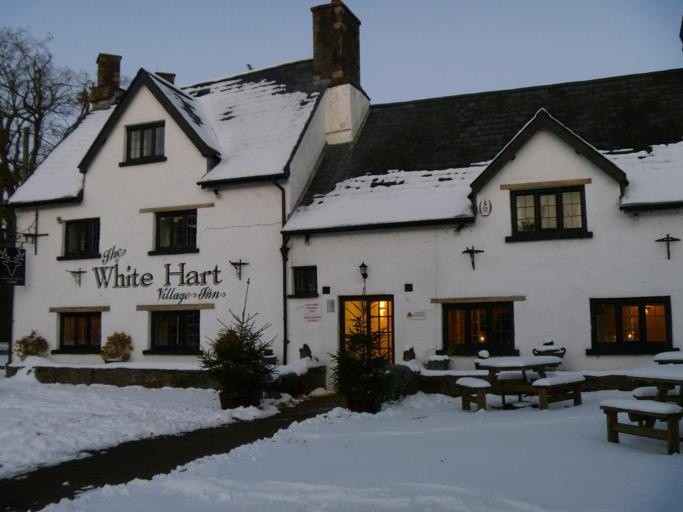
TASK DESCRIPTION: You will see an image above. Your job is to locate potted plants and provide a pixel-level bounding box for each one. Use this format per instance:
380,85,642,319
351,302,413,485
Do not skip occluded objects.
99,332,133,363
13,331,47,361
203,308,278,411
329,325,386,417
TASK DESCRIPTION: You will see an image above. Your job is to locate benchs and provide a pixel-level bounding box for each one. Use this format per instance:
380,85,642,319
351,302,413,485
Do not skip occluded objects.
455,371,585,411
600,387,683,455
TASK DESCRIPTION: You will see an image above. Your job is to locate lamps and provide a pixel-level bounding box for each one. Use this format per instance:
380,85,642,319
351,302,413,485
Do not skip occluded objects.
358,261,367,279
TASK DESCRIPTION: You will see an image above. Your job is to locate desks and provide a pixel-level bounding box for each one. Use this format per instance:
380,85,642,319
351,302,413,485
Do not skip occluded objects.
625,363,683,403
474,356,563,384
